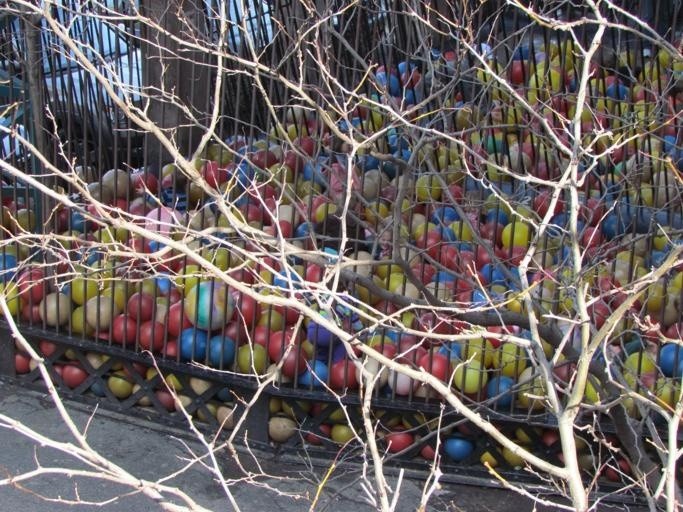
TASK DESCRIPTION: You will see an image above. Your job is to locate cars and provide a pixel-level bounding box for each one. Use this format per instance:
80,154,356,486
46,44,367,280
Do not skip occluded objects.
0,0,391,180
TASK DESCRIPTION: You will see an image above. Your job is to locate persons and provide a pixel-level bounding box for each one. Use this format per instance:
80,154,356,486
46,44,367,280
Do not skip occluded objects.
146,171,189,213
317,212,366,257
